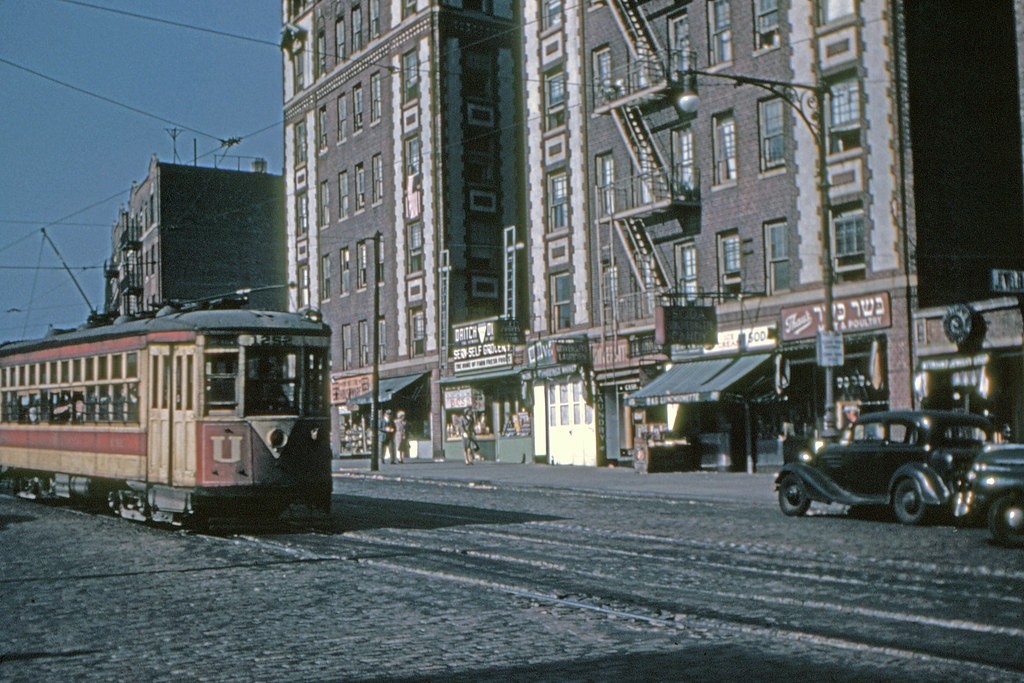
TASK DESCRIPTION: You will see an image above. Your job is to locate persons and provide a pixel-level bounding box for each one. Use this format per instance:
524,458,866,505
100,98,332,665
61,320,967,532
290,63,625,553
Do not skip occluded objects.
378,409,398,465
459,407,478,465
392,411,408,465
1,379,297,425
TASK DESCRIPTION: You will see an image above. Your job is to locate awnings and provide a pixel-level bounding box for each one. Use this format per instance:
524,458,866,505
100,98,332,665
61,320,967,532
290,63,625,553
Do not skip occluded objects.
345,370,431,405
623,351,775,407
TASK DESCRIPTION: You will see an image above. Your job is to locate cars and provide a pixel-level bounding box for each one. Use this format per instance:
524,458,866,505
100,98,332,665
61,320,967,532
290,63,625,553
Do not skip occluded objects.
952,444,1024,548
775,408,1002,526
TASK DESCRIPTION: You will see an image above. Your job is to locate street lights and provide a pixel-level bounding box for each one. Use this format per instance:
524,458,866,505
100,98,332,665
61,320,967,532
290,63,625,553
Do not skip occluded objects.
670,66,842,449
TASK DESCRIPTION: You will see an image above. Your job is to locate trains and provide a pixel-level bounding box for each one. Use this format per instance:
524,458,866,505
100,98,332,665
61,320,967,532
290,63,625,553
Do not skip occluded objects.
0,283,335,531
